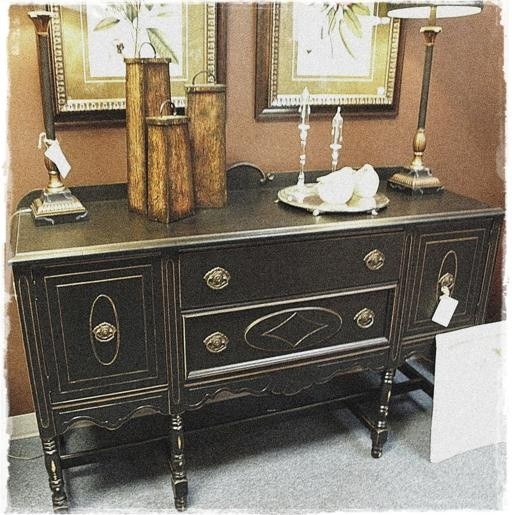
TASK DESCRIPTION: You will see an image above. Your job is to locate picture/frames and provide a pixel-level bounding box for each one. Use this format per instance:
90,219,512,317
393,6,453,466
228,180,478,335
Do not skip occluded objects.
253,1,408,123
32,0,228,130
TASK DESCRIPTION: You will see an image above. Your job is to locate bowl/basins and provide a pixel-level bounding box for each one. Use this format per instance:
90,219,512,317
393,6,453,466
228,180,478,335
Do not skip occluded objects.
316,164,379,205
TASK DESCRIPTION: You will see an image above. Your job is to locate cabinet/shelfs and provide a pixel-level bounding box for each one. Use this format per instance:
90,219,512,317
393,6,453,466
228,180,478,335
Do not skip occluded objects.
7,161,505,514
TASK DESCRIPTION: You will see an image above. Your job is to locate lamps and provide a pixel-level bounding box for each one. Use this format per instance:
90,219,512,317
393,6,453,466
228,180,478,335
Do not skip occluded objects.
29,5,89,227
386,0,483,195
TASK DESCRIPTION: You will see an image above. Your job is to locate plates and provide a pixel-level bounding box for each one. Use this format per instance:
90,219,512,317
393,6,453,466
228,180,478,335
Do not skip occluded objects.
276,183,390,215
